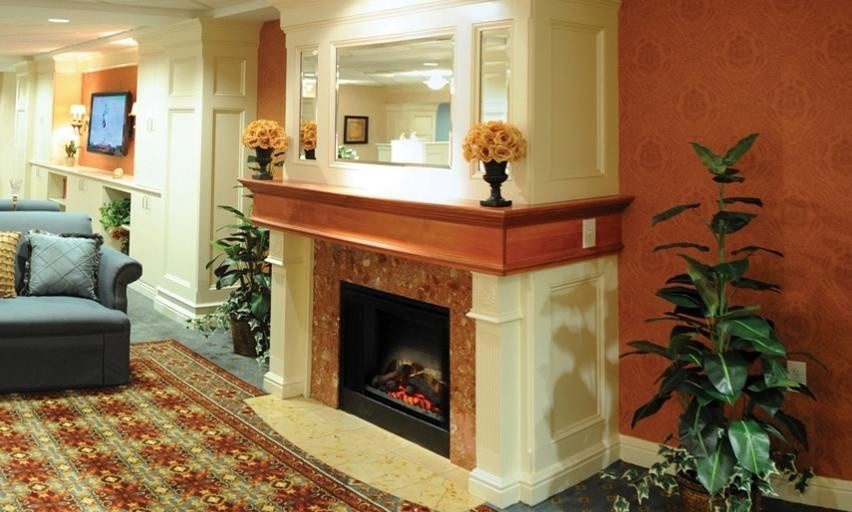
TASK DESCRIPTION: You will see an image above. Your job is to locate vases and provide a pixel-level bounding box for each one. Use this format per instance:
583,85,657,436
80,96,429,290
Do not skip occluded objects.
253,146,274,179
480,160,513,207
304,149,316,160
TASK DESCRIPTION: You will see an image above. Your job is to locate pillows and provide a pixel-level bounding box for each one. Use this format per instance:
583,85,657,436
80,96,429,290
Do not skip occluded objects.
0,229,106,302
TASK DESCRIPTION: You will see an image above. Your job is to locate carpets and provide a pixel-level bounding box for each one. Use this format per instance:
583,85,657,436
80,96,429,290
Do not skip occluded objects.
1,338,501,512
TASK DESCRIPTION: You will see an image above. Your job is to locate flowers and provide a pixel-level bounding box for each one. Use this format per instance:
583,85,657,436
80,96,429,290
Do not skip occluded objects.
238,118,289,150
300,119,317,151
462,119,527,163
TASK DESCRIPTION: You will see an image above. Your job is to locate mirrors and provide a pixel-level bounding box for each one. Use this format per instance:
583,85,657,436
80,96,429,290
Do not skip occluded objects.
474,26,512,177
298,47,319,161
336,34,451,168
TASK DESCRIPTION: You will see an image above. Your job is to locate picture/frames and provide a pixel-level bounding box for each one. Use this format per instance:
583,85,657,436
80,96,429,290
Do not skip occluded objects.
343,115,370,145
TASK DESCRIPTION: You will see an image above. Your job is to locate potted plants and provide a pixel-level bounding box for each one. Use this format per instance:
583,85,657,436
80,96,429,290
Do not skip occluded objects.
594,113,831,512
182,152,287,372
64,140,82,166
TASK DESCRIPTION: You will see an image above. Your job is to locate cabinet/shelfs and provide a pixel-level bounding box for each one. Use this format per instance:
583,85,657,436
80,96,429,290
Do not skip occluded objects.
29,164,65,201
33,53,82,162
64,174,125,254
129,189,169,303
132,25,172,190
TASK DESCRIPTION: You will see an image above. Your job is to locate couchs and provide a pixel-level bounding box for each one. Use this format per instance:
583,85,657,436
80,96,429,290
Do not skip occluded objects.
0,211,143,392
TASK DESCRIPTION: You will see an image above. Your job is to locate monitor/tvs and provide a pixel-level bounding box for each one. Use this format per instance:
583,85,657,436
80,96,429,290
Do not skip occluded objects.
87,92,131,156
